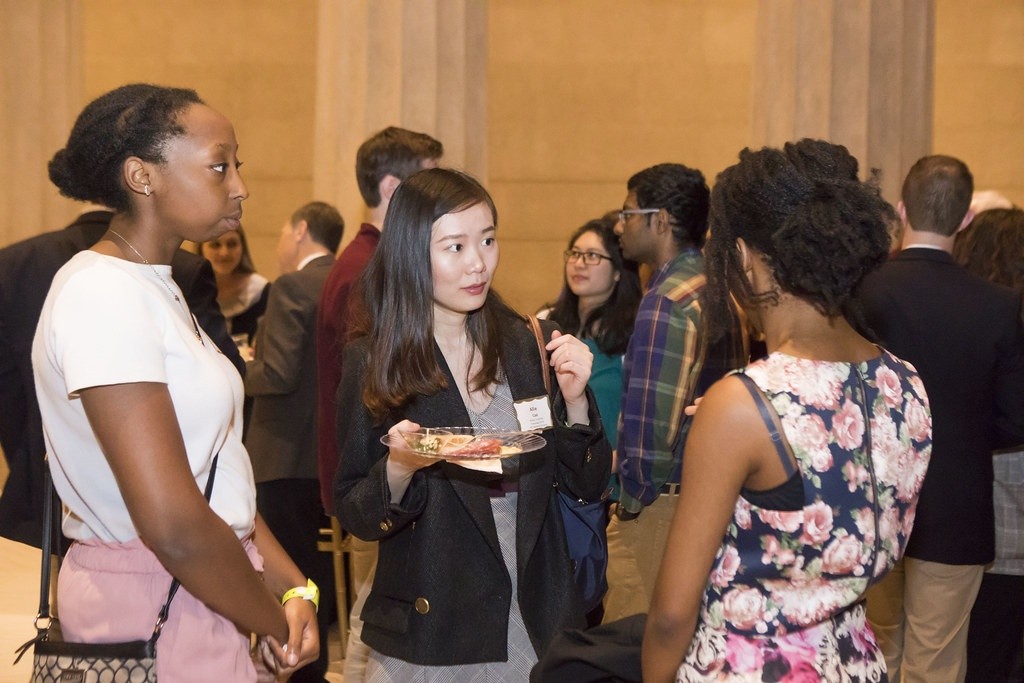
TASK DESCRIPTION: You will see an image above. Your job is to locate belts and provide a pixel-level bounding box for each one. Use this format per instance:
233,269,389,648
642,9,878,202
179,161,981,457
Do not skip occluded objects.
660,485,680,494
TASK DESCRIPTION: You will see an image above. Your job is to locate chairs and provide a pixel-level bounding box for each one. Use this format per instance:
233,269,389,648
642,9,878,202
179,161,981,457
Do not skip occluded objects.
316,511,378,661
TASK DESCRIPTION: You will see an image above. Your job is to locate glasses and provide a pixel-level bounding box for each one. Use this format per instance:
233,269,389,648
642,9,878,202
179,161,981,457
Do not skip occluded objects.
617,209,660,224
561,250,612,265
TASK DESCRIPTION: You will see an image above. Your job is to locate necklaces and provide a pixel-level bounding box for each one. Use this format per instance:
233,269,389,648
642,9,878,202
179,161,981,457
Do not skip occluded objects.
106,223,206,346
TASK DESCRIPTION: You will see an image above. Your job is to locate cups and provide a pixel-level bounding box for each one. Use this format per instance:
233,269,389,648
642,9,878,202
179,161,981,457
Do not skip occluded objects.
231,333,249,350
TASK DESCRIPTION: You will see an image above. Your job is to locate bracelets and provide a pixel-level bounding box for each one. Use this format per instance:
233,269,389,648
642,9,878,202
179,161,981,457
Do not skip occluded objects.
280,578,321,613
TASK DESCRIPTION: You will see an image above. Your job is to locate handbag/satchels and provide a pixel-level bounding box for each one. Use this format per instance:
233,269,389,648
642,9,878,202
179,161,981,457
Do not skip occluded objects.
12,630,158,683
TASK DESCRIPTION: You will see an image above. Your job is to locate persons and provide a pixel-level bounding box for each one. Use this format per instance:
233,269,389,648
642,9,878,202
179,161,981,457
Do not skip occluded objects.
535,137,1024,683
331,165,613,683
32,82,322,683
315,125,443,683
245,201,353,683
0,210,246,558
196,218,272,347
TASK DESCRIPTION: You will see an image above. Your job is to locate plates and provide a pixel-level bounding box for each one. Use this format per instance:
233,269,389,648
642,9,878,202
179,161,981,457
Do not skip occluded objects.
380,427,547,459
